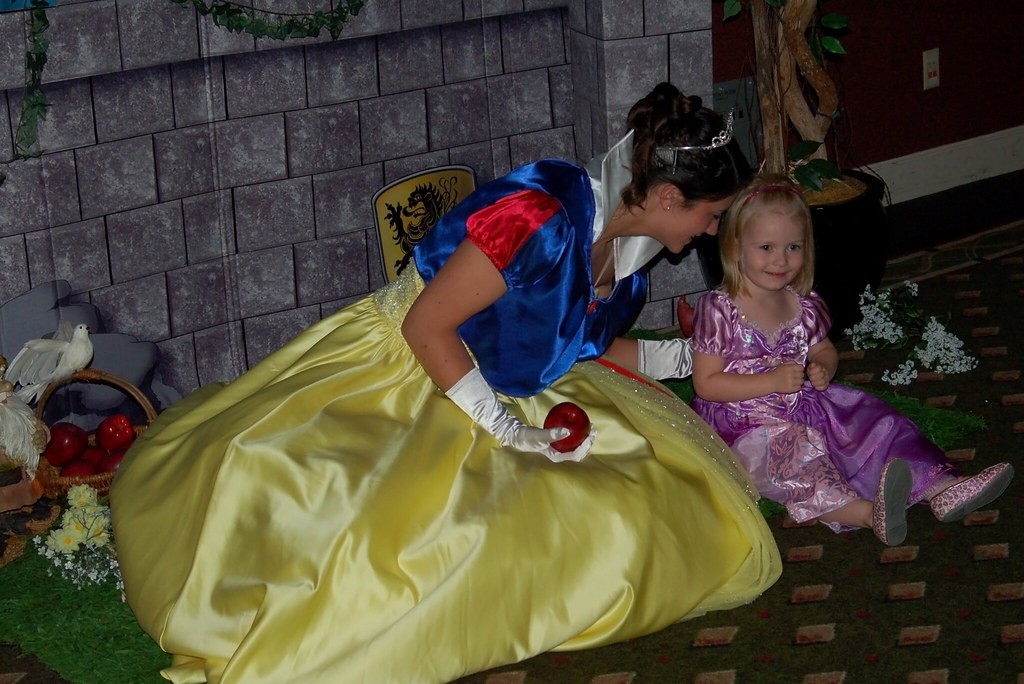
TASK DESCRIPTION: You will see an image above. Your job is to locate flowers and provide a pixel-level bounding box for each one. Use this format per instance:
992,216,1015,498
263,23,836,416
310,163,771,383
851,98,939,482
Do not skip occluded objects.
32,483,137,602
843,278,982,389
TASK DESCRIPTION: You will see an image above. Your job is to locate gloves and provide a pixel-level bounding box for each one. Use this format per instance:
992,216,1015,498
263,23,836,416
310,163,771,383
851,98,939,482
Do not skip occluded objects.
638,338,695,380
444,368,597,463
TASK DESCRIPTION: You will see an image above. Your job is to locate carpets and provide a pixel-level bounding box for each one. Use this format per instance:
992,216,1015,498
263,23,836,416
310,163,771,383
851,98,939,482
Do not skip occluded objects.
0,329,987,684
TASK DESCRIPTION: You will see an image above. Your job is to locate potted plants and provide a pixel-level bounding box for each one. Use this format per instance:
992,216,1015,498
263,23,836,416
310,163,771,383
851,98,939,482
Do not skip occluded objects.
691,0,886,343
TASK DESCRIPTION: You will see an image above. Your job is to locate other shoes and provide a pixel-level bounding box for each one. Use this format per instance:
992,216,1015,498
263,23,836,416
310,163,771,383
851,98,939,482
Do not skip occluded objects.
930,462,1013,522
872,458,913,547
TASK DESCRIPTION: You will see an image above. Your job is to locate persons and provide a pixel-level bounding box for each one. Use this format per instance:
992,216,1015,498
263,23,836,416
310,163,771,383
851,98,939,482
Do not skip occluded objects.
691,173,1015,547
108,80,784,684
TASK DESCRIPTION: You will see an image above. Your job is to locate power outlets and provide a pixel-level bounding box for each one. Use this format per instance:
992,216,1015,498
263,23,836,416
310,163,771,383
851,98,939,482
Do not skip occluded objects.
922,47,940,91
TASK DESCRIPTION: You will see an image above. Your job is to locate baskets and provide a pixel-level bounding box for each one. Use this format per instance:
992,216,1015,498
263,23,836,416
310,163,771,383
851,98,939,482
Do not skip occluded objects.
32,369,157,500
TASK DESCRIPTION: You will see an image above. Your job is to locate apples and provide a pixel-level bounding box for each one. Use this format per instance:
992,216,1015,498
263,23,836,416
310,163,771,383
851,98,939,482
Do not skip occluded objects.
543,402,591,453
44,415,133,477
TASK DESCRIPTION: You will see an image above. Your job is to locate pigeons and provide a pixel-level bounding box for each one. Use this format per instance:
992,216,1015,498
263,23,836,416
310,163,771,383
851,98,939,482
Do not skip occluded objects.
4,319,94,404
